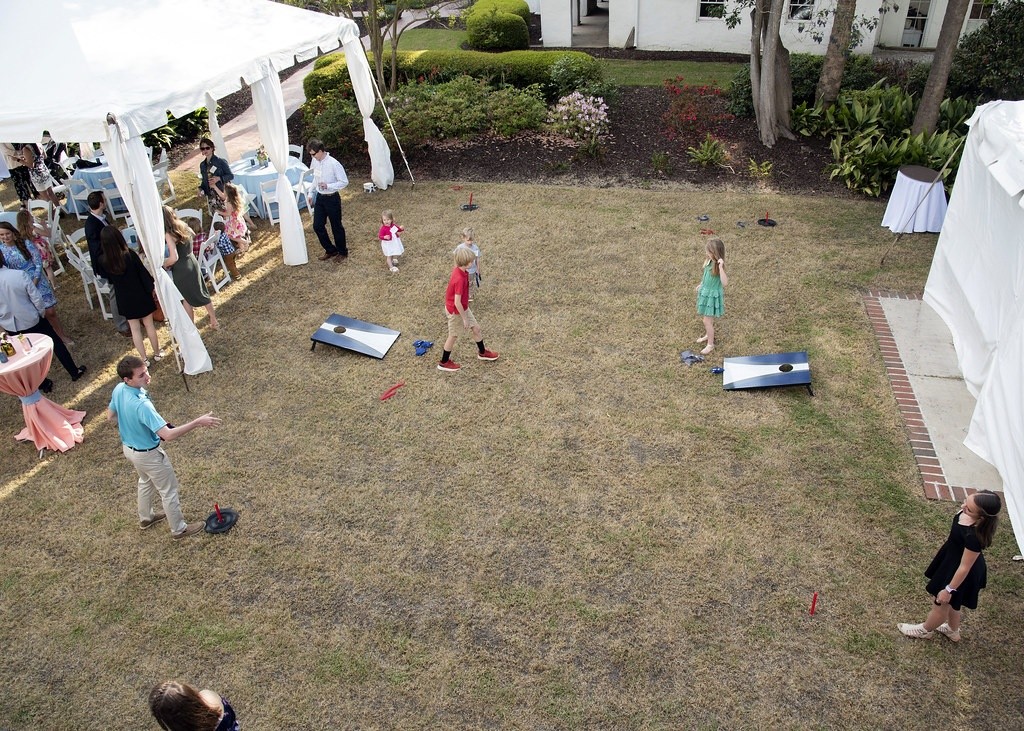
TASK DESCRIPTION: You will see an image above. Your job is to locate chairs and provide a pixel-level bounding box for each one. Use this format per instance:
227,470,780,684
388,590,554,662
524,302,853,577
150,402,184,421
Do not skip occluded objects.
25,145,311,320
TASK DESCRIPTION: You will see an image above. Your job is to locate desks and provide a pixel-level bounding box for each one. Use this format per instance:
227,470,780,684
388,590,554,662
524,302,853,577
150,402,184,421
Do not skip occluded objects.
0,334,87,463
226,155,315,220
126,240,173,289
880,166,948,233
63,156,126,214
0,211,22,232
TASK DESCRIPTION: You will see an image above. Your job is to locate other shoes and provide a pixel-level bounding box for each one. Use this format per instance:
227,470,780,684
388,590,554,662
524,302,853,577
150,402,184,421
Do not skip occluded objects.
388,265,399,273
61,337,76,346
154,350,164,363
119,328,132,337
209,323,220,331
700,344,714,355
468,294,475,302
72,365,86,381
144,359,150,368
697,336,708,343
393,259,399,264
44,379,53,394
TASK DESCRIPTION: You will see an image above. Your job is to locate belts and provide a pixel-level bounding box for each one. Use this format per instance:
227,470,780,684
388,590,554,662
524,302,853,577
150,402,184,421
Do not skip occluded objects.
318,193,335,196
128,443,160,452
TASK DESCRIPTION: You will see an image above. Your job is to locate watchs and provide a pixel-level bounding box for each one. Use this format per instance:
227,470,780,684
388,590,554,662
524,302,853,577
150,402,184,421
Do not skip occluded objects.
946,585,957,594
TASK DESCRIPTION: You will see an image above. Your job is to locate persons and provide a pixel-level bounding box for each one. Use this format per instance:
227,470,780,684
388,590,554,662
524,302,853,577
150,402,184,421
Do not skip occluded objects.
98,226,166,367
197,138,235,216
896,490,1001,643
0,143,60,217
107,355,222,541
188,217,210,275
695,238,728,355
162,204,220,330
213,222,242,280
456,228,482,301
0,220,75,345
378,210,405,271
85,190,144,337
148,680,238,731
209,183,251,254
437,246,500,371
0,250,87,393
16,210,58,292
307,139,350,261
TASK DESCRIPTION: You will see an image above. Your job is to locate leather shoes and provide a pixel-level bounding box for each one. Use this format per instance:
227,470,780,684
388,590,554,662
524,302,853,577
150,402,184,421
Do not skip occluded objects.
333,251,348,263
318,250,337,259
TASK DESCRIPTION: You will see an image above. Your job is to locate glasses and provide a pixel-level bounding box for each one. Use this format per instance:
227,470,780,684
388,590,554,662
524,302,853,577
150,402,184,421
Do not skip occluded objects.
309,151,318,155
200,146,211,150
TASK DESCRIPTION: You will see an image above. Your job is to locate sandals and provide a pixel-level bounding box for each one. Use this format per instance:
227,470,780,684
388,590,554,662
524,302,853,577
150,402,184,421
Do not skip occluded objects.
897,623,932,640
936,622,960,642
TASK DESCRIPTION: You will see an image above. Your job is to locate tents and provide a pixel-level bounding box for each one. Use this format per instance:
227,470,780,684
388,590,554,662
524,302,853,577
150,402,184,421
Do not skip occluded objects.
918,100,1024,562
0,0,395,377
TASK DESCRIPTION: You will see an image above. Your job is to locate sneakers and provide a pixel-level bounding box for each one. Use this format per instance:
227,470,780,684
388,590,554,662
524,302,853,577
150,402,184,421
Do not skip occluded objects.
173,521,206,541
478,350,499,361
437,359,461,372
140,513,166,529
